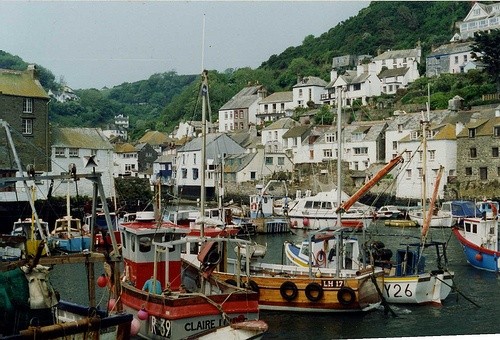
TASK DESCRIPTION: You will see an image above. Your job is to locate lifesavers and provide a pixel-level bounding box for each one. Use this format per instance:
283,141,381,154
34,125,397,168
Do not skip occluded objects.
337,287,356,306
280,281,298,301
283,209,288,217
221,280,260,297
305,282,323,302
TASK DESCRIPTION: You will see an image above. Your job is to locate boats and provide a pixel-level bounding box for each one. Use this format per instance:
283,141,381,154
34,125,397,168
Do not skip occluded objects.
0,14,499,340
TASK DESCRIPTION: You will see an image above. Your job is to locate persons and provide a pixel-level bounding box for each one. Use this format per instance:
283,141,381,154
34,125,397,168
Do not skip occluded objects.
142,276,162,295
